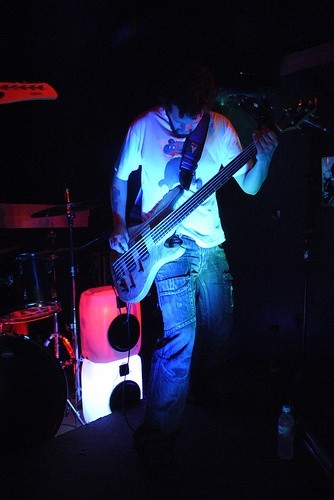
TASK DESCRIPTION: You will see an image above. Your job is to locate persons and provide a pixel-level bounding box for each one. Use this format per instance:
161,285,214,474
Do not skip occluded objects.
108,59,280,447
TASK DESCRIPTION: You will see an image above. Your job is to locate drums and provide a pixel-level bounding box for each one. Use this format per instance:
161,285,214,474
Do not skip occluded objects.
1,330,69,473
1,302,69,360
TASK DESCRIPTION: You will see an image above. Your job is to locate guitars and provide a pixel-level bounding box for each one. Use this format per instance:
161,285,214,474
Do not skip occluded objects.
0,81,60,107
110,96,320,301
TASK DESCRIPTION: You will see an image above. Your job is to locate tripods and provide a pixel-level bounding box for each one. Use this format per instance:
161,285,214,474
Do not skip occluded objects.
44,228,74,360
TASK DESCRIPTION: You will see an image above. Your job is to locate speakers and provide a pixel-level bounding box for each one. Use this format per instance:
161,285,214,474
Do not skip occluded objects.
80,355,143,425
80,285,141,363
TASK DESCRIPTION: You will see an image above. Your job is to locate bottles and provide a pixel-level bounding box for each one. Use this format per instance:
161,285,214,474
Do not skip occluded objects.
277,406,295,461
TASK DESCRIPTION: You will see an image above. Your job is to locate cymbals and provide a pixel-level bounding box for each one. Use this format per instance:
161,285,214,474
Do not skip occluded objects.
12,244,89,260
31,200,105,218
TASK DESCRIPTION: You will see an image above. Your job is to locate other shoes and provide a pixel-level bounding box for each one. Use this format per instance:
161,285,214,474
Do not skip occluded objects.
137,425,185,470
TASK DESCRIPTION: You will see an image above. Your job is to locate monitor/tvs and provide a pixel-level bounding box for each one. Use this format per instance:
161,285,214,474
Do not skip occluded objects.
310,148,334,210
